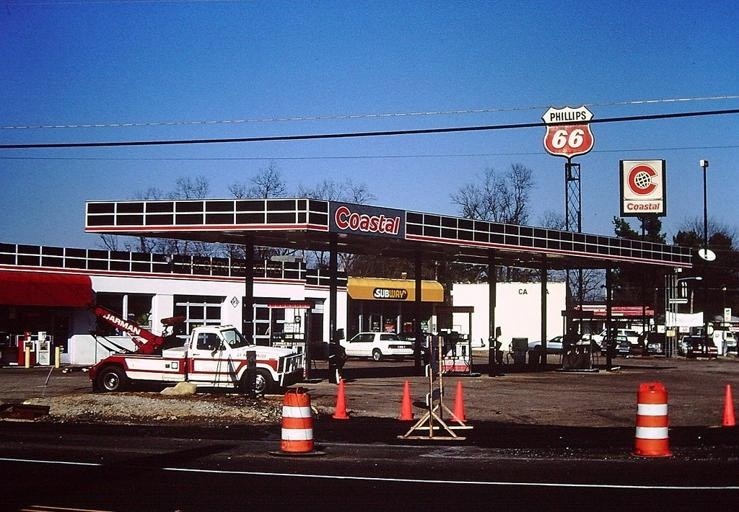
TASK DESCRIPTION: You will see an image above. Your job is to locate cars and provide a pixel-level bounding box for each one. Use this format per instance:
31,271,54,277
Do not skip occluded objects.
339,330,415,362
526,326,739,360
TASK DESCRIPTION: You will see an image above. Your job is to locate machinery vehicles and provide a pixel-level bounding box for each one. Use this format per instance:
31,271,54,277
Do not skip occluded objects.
81,302,306,391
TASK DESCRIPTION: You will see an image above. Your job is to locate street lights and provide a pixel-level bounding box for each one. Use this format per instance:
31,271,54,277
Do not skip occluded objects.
698,158,712,354
674,276,703,355
721,286,728,307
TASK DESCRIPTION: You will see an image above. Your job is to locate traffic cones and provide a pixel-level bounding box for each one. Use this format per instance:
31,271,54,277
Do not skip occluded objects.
329,377,350,420
397,380,415,421
448,379,469,424
717,382,738,428
